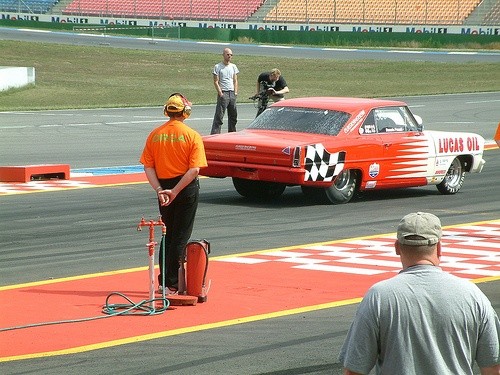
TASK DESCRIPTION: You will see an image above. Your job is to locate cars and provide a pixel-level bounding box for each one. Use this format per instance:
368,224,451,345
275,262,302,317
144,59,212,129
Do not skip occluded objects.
196,95,485,206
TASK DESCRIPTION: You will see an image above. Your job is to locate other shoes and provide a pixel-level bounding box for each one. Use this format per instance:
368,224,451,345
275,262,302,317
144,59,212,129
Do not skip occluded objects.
158,285,175,295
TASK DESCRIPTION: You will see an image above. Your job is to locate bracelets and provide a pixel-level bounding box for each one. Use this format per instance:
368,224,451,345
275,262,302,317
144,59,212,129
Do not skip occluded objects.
155,187,162,191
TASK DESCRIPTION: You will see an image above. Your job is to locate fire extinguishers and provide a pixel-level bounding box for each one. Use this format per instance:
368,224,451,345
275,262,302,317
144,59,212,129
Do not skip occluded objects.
182,239,211,302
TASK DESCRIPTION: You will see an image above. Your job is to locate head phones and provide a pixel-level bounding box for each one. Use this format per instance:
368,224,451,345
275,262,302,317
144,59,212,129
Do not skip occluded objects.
164,93,191,118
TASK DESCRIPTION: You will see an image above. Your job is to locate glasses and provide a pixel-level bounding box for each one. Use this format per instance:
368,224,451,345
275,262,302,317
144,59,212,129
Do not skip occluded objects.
227,54,232,56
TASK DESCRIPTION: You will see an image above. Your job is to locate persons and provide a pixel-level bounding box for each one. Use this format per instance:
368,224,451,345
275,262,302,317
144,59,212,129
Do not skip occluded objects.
210,47,239,136
338,212,500,375
255,69,289,119
140,94,208,295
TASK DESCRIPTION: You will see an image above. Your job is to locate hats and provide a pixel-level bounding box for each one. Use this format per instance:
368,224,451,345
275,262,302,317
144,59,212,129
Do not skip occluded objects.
167,95,192,113
397,212,441,246
